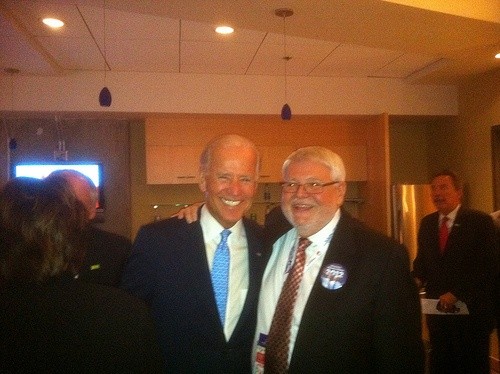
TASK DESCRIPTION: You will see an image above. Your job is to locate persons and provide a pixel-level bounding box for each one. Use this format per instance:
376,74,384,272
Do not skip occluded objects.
0,170,160,374
171,146,425,374
116,134,276,374
409,170,500,374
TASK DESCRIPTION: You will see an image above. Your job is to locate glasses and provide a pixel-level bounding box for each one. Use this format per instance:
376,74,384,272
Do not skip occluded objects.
279,179,340,194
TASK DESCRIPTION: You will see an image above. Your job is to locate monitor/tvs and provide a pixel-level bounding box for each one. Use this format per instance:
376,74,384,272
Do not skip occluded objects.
11,159,106,221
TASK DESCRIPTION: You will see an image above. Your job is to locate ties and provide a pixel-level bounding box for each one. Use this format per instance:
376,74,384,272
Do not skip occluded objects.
210,228,231,331
439,216,449,255
262,237,312,374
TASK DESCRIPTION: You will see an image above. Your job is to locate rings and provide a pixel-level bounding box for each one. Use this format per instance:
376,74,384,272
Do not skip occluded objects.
443,305,449,309
